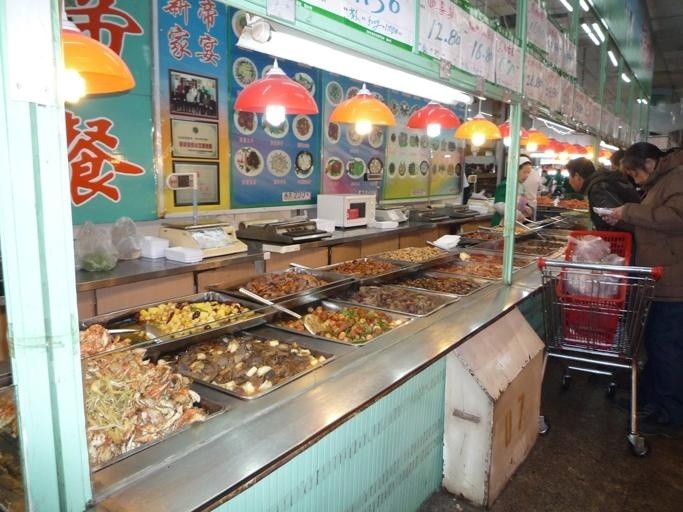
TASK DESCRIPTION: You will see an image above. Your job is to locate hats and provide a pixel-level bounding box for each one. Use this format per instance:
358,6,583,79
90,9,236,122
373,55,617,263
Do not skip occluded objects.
517,155,532,166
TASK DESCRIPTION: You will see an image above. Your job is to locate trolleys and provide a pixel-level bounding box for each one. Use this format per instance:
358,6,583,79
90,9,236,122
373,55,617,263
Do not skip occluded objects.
536,255,663,458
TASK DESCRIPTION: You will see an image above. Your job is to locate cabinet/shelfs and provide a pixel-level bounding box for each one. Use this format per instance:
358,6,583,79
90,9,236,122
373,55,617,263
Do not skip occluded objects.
2,220,492,388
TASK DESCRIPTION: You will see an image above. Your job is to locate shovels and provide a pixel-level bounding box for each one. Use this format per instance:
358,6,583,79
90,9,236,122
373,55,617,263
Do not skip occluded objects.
426,240,471,261
239,288,326,337
515,219,546,240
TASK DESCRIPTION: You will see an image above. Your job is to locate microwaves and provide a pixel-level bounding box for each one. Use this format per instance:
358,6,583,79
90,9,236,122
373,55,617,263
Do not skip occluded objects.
316,194,376,228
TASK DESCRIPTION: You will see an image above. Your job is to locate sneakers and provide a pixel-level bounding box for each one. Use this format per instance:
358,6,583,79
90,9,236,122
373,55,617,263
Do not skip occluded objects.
603,384,681,440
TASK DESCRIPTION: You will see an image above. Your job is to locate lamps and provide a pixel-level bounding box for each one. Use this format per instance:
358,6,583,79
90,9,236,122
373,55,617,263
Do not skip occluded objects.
329,83,394,135
235,58,319,127
454,96,503,146
235,13,476,105
408,100,461,138
497,115,620,166
61,0,135,104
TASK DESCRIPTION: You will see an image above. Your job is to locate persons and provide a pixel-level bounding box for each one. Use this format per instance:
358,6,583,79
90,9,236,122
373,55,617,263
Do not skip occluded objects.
542,169,553,196
519,153,541,222
608,149,647,199
597,139,682,441
488,154,533,228
564,156,642,388
554,167,563,196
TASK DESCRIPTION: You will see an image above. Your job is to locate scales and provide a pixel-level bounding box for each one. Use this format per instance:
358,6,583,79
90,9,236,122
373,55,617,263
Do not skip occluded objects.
160,172,249,259
